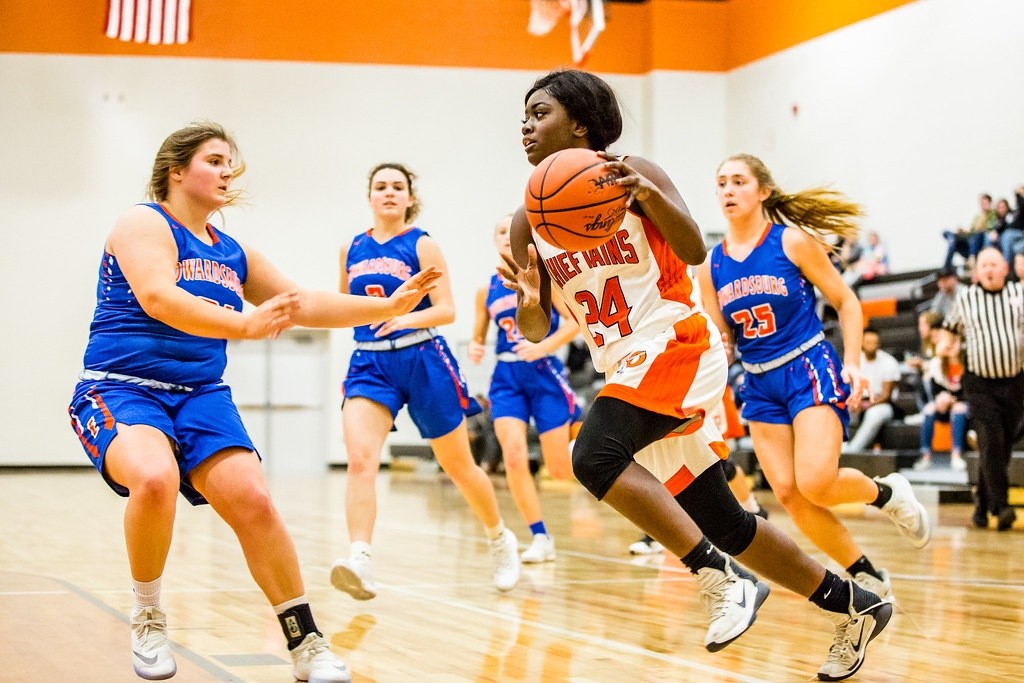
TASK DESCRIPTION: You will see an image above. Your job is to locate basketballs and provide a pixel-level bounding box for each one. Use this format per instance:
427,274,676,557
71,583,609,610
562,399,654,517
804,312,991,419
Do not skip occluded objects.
524,147,628,253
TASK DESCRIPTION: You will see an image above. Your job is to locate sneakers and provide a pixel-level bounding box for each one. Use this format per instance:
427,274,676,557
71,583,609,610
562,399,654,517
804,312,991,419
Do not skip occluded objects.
873,472,930,548
130,607,177,680
487,529,520,591
817,580,892,681
851,568,896,603
330,549,376,600
288,631,349,683
690,552,770,652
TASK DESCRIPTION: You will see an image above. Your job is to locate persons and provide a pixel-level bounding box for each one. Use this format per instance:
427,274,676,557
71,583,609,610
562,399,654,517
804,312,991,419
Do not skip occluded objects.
328,163,521,600
468,186,1023,556
698,155,930,602
69,126,443,683
467,214,577,564
494,71,893,681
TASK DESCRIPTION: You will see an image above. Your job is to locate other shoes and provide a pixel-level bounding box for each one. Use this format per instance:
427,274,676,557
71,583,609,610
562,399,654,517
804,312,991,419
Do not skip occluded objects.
997,507,1016,530
973,492,988,527
522,533,554,563
630,534,665,555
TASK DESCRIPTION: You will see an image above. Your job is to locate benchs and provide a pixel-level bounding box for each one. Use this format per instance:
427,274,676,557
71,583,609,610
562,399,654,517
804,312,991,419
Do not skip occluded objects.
840,453,919,482
390,446,436,471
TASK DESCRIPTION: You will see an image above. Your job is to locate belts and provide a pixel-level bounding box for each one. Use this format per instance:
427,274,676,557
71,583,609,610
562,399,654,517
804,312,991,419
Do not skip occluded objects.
741,330,825,374
496,351,523,362
354,327,438,351
